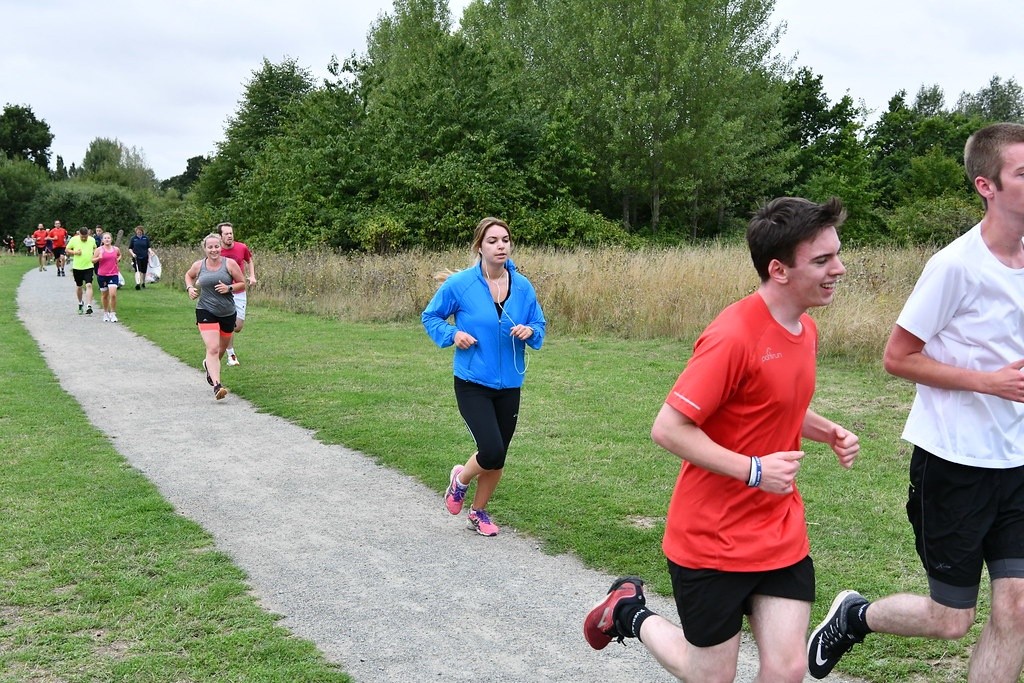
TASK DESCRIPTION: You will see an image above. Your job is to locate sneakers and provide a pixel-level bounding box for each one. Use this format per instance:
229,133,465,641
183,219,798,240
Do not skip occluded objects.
78,299,83,315
807,589,869,680
103,311,108,322
109,311,118,323
444,463,468,515
584,573,646,649
226,355,240,367
467,502,498,537
203,359,216,386
86,307,93,315
214,382,227,401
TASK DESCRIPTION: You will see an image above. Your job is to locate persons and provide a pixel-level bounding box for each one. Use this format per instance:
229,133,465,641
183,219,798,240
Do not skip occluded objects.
65,227,98,315
45,229,53,263
421,217,547,536
47,219,68,276
4,235,14,254
30,235,36,255
217,222,256,366
94,225,104,275
66,235,71,265
32,224,47,272
24,235,32,256
129,227,153,289
185,234,246,400
92,233,122,322
807,121,1024,683
88,228,93,237
583,196,859,683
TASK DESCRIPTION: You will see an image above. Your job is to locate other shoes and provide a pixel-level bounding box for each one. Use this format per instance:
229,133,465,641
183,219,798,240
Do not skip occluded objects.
42,266,48,272
135,284,141,290
39,265,44,273
61,270,65,277
58,270,61,278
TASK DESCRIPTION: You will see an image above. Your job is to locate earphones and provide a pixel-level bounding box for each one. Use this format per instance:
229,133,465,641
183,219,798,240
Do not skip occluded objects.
478,247,483,254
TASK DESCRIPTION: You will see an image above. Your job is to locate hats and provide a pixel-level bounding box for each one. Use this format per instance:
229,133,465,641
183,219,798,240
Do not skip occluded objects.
38,223,44,229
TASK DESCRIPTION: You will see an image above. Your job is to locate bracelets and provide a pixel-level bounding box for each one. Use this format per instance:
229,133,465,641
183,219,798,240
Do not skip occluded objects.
529,327,534,331
186,286,190,292
747,456,761,486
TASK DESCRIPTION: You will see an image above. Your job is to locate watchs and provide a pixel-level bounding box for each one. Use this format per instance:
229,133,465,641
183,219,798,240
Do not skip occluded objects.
228,285,233,292
